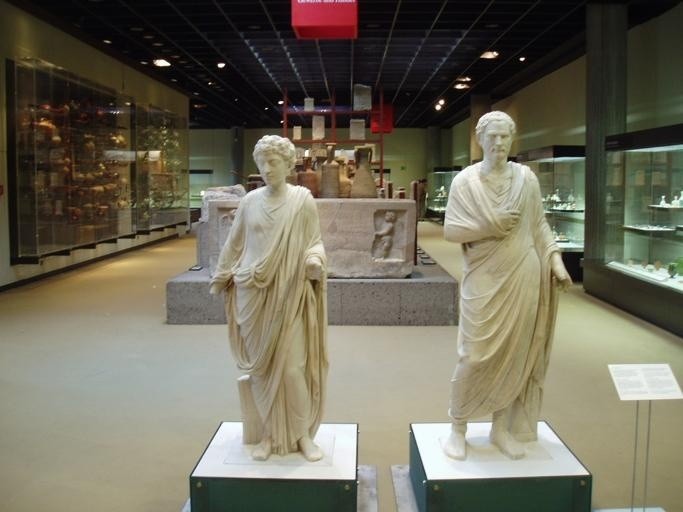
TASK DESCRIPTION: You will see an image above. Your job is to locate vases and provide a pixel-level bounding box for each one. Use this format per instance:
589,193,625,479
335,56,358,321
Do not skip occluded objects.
290,140,379,202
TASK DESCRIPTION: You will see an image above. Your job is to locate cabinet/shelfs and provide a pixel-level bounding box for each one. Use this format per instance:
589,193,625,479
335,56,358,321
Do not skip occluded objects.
540,195,585,221
431,187,448,203
281,105,383,189
615,192,682,244
14,56,137,257
132,104,188,235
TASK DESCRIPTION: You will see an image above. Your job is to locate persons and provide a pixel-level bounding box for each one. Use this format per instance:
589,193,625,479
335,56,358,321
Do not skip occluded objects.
445,110,572,458
376,212,395,256
213,135,330,461
